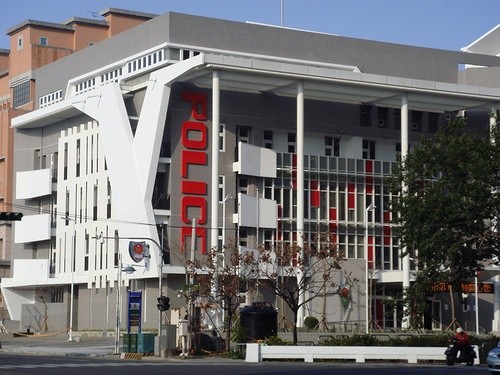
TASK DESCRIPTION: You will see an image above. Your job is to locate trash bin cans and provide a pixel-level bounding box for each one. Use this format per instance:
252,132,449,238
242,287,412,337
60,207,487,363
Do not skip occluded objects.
123,332,157,356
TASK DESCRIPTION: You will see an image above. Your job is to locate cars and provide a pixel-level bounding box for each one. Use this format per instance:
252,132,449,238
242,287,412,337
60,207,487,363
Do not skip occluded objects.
486,342,500,371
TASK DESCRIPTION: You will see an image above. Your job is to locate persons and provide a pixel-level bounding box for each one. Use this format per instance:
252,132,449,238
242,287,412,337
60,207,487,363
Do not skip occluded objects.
449,328,469,359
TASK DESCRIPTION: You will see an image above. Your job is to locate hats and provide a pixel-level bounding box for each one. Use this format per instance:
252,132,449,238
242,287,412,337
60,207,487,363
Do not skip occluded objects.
456,327,463,333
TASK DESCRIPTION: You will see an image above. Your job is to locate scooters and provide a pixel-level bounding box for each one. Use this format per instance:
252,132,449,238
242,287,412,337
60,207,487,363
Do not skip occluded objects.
444,340,477,366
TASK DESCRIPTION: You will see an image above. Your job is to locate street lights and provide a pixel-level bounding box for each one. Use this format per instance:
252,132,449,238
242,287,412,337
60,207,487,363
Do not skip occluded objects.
100,222,165,357
61,215,75,341
365,203,376,334
222,194,233,321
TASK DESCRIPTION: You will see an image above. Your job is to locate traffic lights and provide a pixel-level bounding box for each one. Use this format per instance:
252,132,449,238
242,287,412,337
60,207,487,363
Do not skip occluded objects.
0,212,23,221
157,296,170,311
